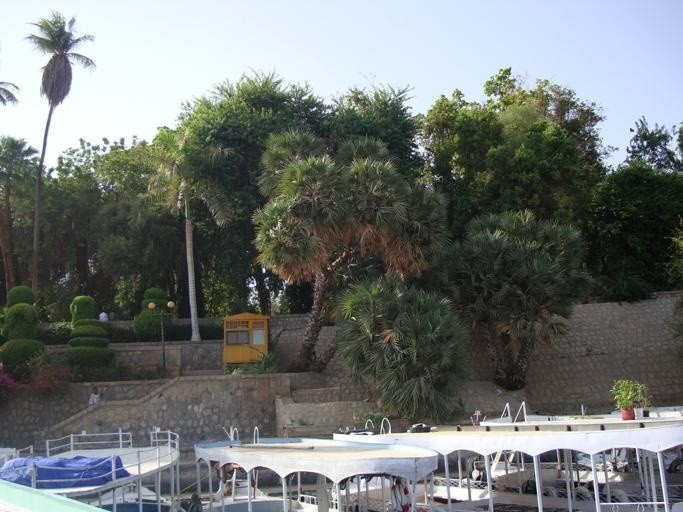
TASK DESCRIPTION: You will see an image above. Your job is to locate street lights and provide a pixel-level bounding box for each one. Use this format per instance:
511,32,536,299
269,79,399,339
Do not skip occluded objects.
147,300,178,378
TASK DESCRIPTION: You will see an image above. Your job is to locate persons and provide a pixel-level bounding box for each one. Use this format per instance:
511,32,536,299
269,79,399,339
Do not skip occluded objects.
108,307,118,320
88,387,99,405
97,309,108,321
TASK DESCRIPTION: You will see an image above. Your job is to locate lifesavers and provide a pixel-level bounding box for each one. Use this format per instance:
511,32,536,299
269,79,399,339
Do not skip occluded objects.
393,478,411,511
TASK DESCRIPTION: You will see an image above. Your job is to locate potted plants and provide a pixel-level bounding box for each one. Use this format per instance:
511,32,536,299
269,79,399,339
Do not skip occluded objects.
609,378,651,420
